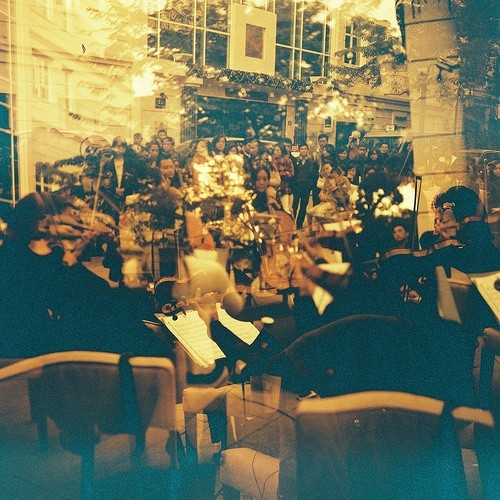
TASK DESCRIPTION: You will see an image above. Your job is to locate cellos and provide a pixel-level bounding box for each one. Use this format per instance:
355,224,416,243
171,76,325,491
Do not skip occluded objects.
255,206,301,306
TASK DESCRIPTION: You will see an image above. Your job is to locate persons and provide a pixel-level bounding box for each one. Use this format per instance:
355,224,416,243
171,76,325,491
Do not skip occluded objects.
0,191,156,360
490,160,499,208
41,132,414,332
400,185,500,318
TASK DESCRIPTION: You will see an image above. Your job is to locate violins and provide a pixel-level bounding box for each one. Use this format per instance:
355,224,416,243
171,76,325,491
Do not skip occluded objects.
153,272,207,301
362,239,460,271
28,224,81,256
435,210,500,234
